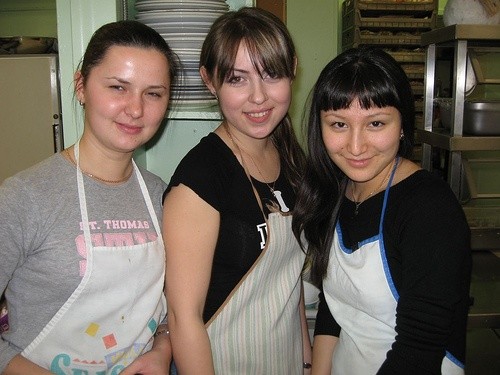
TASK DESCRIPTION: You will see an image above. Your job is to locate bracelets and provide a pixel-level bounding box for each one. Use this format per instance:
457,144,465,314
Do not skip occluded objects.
151,330,171,335
304,361,310,368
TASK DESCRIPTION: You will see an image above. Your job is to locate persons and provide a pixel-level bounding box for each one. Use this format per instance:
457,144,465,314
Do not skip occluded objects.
161,6,317,375
292,44,474,375
0,15,183,375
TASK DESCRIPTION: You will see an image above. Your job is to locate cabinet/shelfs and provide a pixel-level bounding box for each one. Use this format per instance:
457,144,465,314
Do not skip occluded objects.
56,0,341,183
342,1,500,329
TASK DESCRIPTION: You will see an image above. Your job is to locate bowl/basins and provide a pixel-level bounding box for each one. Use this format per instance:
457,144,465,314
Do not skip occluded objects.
10,35,57,54
438,101,500,136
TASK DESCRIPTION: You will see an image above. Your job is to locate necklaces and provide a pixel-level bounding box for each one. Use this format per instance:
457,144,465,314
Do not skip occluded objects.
62,146,137,183
343,164,391,213
228,124,292,219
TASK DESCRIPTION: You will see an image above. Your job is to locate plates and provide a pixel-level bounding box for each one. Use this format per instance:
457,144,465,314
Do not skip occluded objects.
133,1,231,108
